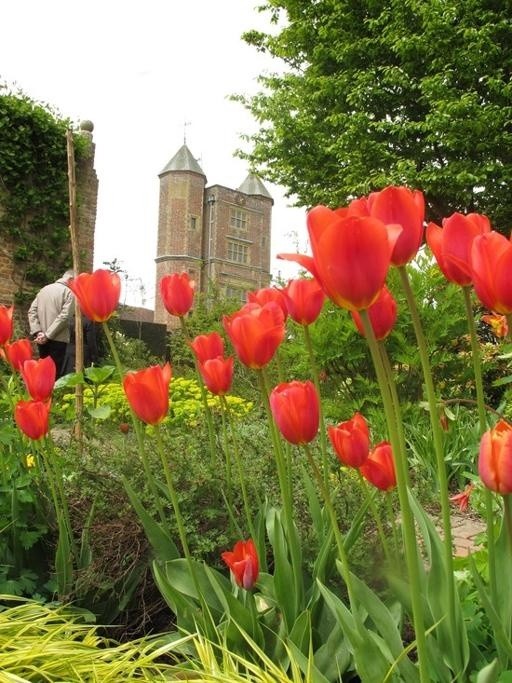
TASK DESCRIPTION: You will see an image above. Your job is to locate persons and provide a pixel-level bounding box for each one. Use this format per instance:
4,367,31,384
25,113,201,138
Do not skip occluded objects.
27,270,79,379
